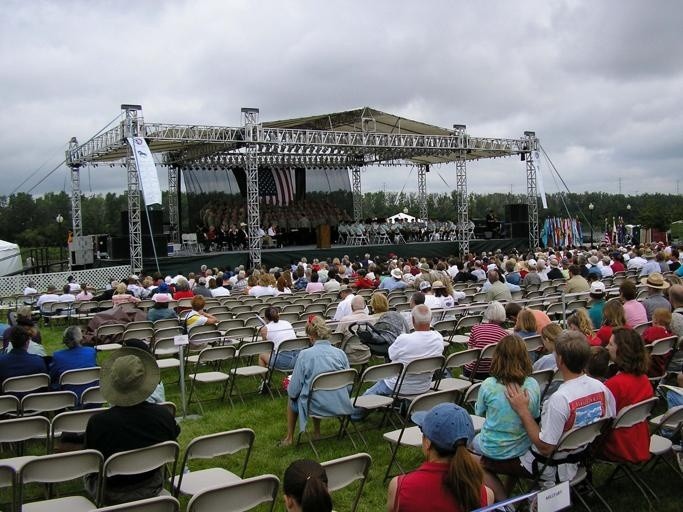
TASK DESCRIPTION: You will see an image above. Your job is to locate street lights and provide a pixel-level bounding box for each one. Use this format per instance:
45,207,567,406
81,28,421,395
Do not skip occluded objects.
589,201,595,248
55,214,67,273
626,204,631,224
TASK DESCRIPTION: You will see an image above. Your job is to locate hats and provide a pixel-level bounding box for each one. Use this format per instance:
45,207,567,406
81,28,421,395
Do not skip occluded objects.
588,256,610,265
48,285,56,293
99,347,160,406
528,254,559,268
419,280,446,290
641,273,670,289
131,275,139,279
391,268,402,279
657,242,665,247
337,285,352,299
411,402,474,450
643,249,656,257
155,275,187,302
590,281,605,294
418,263,432,271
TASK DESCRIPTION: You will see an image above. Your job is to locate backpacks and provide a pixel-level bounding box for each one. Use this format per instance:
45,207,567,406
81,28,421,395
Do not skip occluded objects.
178,312,199,335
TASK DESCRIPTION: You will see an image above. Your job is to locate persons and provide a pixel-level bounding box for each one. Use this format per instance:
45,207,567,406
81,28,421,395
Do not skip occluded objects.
0,210,683,512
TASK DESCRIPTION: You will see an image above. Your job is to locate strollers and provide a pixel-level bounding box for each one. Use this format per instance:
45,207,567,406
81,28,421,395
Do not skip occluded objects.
346,310,410,363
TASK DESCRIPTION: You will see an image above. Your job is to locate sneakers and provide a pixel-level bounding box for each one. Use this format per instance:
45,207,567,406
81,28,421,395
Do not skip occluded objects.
576,484,594,498
259,379,269,394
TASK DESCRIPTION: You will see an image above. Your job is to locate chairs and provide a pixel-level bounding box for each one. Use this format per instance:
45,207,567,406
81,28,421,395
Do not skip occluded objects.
337,222,476,246
180,231,283,256
